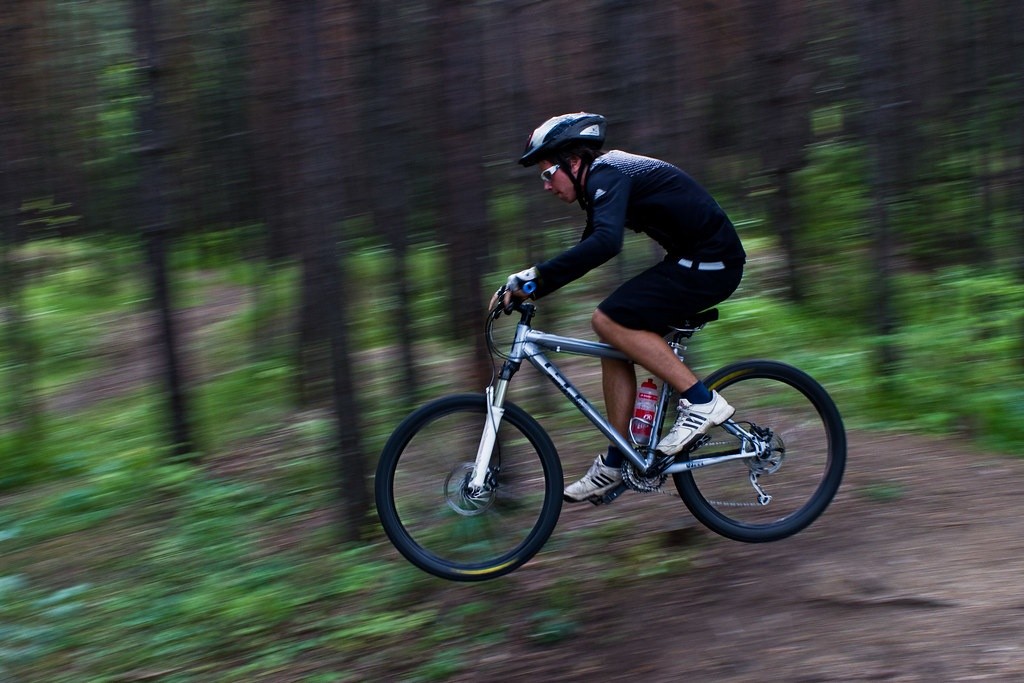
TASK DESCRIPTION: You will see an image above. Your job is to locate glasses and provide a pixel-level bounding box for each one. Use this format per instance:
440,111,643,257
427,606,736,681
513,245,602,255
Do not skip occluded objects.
540,156,571,183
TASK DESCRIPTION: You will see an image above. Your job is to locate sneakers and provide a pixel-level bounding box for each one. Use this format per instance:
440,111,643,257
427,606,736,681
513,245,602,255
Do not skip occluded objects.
655,388,736,457
563,453,624,503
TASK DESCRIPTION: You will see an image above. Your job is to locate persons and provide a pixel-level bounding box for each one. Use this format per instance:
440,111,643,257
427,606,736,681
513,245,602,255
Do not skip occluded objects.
488,112,746,502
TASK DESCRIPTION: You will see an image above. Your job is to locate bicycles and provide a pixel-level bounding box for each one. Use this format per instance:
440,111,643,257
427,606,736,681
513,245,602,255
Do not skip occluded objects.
373,281,848,583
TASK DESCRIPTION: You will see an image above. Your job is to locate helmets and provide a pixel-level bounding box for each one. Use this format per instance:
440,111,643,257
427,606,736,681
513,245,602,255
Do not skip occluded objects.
518,111,608,168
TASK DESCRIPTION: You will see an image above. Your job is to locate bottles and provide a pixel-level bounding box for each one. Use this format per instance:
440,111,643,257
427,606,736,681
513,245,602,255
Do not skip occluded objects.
628,379,659,444
